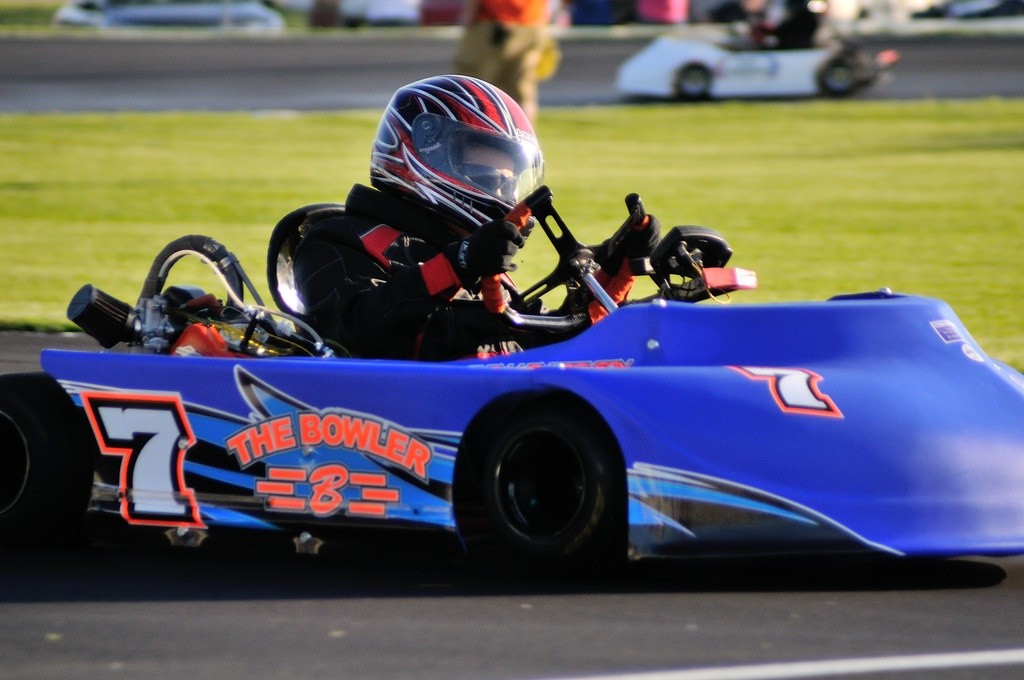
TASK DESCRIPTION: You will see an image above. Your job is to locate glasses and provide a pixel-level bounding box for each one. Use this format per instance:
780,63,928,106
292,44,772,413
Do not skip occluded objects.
454,161,520,202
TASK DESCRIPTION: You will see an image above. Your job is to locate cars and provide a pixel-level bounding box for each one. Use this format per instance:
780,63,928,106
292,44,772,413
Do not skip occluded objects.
52,0,287,31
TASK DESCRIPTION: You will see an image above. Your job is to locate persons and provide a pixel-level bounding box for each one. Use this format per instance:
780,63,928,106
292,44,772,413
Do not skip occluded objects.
300,0,1024,146
294,73,660,362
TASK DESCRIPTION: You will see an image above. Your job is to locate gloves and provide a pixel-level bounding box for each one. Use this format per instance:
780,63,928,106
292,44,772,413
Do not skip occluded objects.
609,214,661,264
445,219,526,290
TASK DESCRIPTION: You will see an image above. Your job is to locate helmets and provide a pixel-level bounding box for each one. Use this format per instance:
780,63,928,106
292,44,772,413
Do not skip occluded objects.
370,74,544,241
